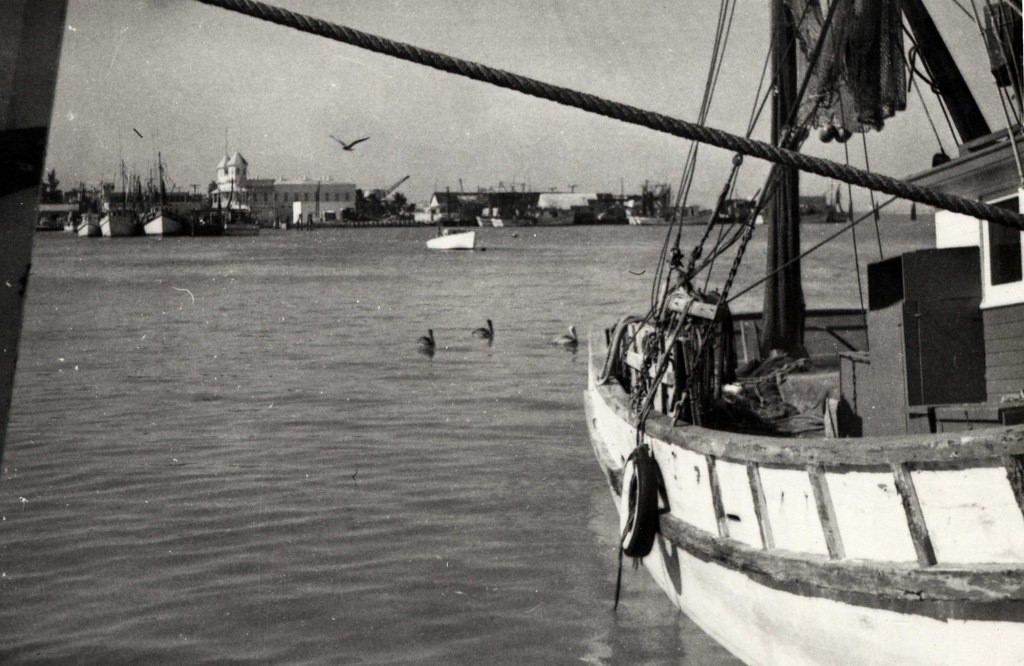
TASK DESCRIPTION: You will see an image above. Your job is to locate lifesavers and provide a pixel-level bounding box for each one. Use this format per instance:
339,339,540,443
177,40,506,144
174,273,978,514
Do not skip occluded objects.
622,452,658,556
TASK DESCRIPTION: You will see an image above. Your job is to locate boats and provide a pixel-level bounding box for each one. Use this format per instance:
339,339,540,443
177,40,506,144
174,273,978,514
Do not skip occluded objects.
475,207,574,228
425,226,475,250
626,205,712,225
749,202,851,225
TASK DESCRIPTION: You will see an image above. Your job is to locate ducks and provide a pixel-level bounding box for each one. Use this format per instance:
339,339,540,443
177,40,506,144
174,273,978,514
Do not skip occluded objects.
417,329,435,346
471,318,494,337
551,325,579,346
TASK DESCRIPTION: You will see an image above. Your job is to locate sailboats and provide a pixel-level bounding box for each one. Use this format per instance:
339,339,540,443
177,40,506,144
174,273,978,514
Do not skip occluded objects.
582,1,1023,665
63,152,262,237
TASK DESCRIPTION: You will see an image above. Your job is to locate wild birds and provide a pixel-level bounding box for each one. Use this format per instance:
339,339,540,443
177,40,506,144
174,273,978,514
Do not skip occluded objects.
329,134,370,152
